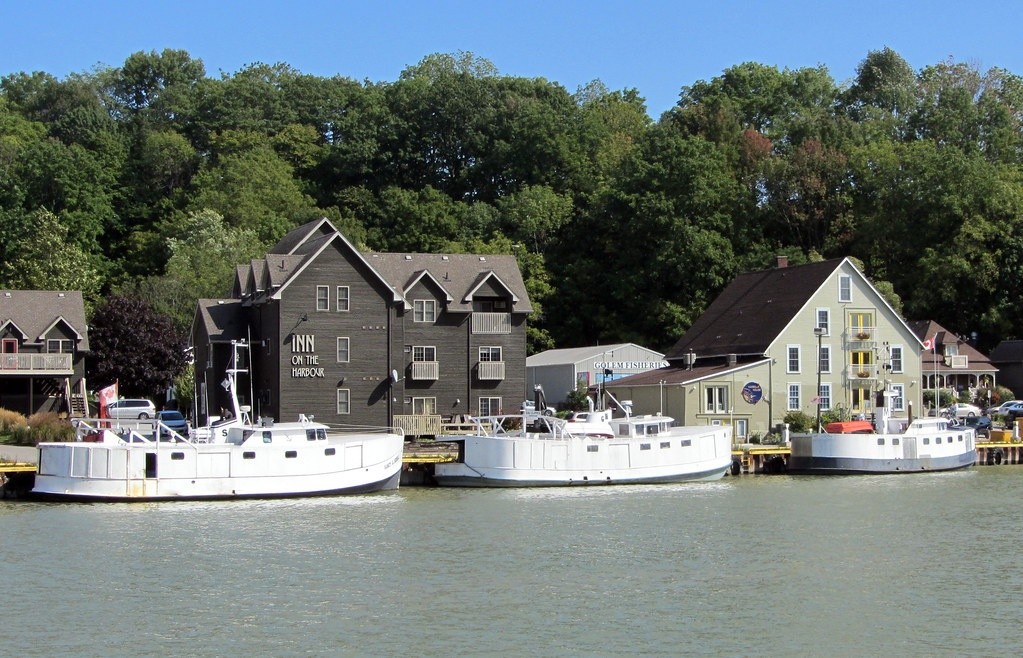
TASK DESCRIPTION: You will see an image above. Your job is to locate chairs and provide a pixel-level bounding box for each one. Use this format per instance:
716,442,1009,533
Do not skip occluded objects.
451,413,478,430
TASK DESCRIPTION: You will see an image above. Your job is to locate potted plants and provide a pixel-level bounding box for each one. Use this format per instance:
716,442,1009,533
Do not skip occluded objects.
857,372,870,378
857,333,870,340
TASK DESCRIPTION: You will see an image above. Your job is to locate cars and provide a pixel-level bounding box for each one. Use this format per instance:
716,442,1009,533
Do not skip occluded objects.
563,412,590,422
929,397,1023,439
526,400,557,424
152,410,188,435
105,398,156,419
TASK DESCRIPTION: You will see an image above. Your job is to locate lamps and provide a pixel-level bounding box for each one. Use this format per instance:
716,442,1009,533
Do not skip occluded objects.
240,338,245,343
456,399,460,403
912,381,916,385
230,339,237,344
392,369,398,384
300,311,308,321
341,377,347,382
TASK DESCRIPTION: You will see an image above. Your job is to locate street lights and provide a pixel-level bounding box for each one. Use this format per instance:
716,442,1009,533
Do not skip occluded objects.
813,327,827,432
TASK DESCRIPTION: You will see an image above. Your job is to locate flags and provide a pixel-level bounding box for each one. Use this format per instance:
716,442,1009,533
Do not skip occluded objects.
810,395,821,404
98,381,118,406
922,337,934,351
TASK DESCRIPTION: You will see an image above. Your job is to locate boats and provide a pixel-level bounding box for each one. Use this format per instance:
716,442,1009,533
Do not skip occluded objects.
786,416,977,473
26,324,404,498
431,380,734,488
